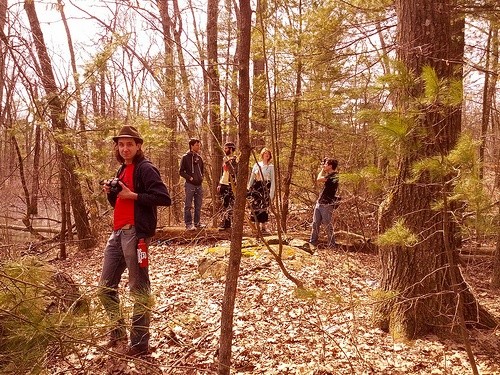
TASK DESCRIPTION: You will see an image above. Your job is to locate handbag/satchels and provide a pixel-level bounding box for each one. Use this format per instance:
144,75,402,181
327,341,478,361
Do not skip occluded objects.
333,197,341,209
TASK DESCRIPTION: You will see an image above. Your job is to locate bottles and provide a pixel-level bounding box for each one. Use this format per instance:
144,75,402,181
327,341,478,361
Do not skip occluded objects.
137,239,148,267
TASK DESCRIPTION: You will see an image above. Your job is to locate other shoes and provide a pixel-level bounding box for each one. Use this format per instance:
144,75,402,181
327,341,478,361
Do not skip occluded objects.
117,343,149,358
186,224,197,231
219,218,231,230
261,230,269,234
195,222,208,228
93,338,128,355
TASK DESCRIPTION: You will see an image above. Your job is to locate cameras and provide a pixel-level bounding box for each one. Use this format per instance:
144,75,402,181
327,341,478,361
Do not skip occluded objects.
107,177,123,193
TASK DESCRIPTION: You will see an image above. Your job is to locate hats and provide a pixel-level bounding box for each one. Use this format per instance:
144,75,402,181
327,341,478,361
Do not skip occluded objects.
112,126,143,145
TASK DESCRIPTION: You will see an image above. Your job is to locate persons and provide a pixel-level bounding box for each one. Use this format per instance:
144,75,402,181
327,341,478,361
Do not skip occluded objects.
247,147,275,232
217,141,238,229
179,138,206,231
96,125,171,357
310,157,340,249
